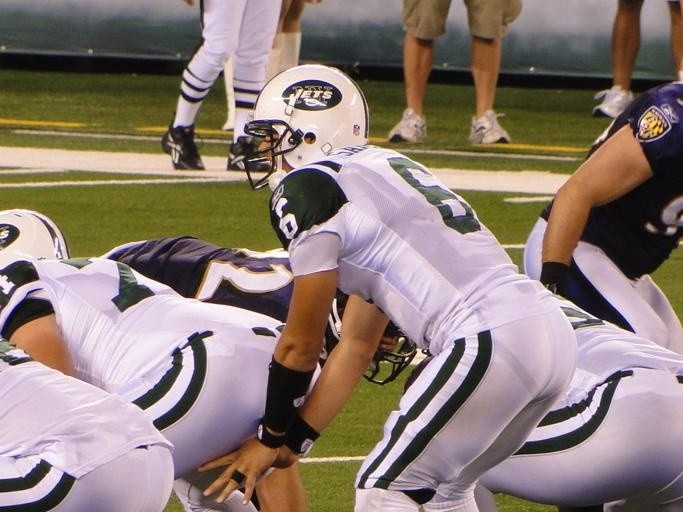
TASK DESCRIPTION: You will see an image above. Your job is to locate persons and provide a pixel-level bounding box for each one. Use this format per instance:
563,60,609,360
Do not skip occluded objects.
522,81,683,357
97,234,416,511
0,207,321,512
158,0,281,177
320,276,683,511
196,59,579,511
188,0,320,138
385,1,523,147
0,336,175,512
592,0,683,120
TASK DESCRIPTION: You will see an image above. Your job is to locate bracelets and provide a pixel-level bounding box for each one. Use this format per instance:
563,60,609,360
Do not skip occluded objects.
283,416,320,457
540,261,574,299
256,422,285,448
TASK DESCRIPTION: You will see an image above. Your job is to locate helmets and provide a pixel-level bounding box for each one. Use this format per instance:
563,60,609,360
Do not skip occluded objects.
237,60,371,193
318,285,418,386
0,204,71,273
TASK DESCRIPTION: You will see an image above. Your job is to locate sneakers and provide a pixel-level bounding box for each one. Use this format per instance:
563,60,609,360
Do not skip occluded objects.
160,120,206,172
386,106,427,144
590,83,635,121
225,135,277,173
467,108,511,144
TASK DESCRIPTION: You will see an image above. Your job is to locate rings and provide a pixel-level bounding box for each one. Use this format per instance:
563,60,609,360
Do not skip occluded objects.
230,470,244,483
